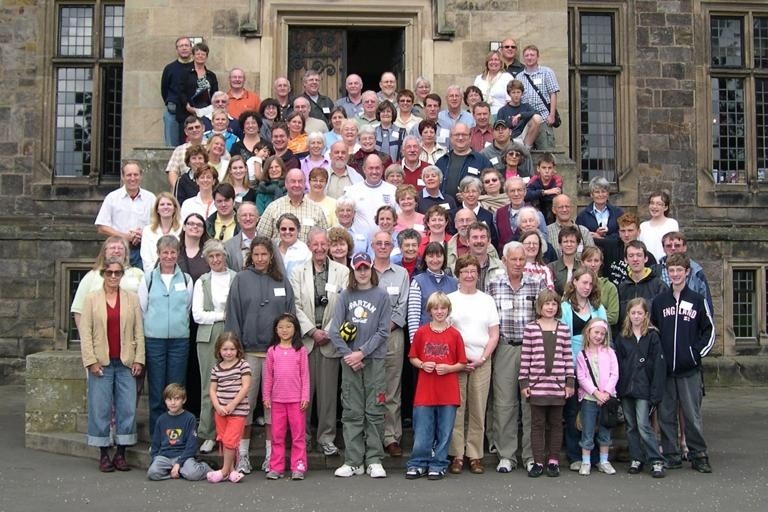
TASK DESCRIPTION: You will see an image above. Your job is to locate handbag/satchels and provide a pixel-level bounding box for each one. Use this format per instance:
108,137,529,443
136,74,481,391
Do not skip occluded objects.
546,103,561,128
602,397,625,428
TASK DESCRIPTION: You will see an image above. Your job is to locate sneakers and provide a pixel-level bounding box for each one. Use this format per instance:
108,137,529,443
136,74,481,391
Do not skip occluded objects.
316,441,484,480
495,457,712,478
199,439,305,482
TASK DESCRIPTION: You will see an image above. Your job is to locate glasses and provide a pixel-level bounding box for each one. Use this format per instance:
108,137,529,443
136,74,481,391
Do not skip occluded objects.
485,178,496,183
500,46,517,50
666,244,680,247
104,270,122,277
219,225,226,241
281,227,294,231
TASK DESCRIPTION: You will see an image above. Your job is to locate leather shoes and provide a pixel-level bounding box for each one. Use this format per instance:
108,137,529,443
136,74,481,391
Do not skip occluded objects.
99,454,114,472
112,454,128,471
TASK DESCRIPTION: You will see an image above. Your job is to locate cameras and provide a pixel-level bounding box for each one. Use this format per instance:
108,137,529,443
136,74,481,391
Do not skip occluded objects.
315,295,328,307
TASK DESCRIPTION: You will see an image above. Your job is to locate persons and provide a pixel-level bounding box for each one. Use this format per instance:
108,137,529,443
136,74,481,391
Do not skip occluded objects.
160,37,218,146
475,38,560,149
96,163,155,269
71,236,150,471
135,68,716,478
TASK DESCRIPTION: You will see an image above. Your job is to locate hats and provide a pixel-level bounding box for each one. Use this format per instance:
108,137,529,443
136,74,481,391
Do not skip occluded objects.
494,120,508,129
351,253,372,270
